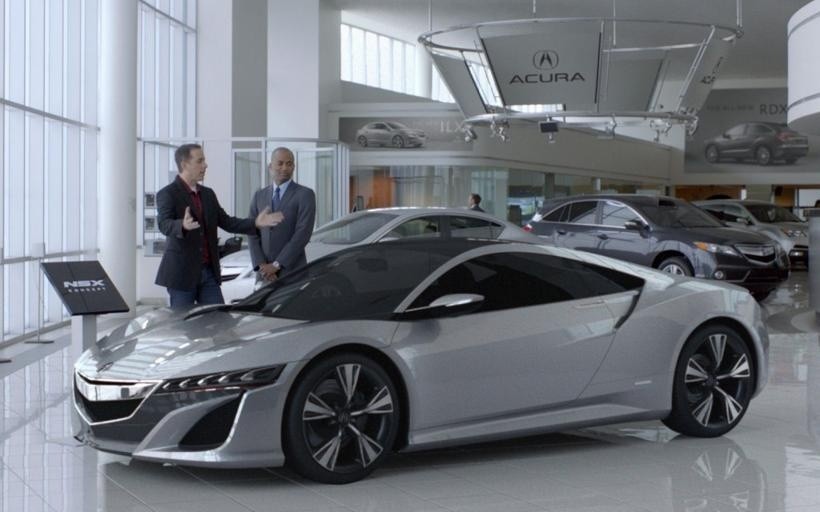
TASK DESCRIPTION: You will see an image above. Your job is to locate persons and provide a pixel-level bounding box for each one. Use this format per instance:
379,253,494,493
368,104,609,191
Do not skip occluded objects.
248,147,317,286
156,144,285,306
466,193,485,212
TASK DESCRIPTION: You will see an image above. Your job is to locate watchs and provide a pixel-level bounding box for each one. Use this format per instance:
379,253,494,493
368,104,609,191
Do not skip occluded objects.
272,261,281,271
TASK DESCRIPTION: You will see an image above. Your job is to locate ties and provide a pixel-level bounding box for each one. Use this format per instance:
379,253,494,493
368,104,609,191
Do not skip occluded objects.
272,186,281,212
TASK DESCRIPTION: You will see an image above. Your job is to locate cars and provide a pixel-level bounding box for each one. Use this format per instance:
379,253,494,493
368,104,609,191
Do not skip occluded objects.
525,196,794,304
692,198,812,272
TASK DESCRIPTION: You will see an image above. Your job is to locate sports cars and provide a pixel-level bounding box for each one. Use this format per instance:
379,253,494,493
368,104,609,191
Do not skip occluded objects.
72,237,776,486
220,207,556,304
